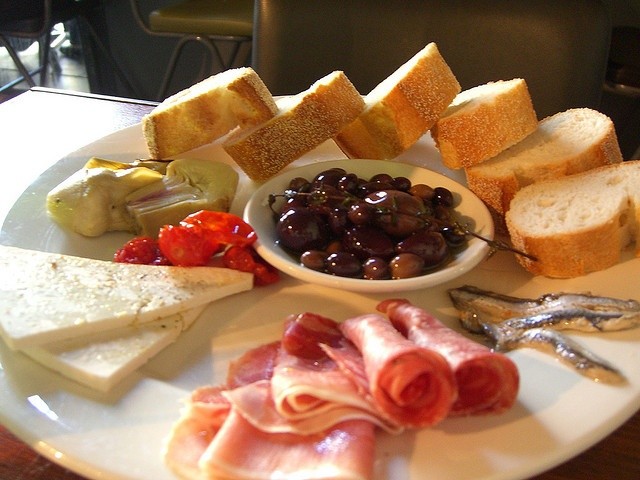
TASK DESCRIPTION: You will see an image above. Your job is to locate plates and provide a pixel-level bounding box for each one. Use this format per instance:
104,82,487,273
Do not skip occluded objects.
242,158,495,293
0,96,640,480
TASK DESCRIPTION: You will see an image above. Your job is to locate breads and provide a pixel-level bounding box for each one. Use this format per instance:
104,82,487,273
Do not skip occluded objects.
466,107,624,235
504,160,640,278
430,78,538,170
335,40,461,164
140,66,278,162
224,69,366,183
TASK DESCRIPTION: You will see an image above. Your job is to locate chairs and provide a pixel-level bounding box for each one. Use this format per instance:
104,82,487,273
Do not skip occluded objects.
254,2,614,120
0,0,139,98
130,1,253,102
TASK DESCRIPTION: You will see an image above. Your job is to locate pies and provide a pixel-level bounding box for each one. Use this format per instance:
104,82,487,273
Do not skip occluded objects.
25,301,211,393
0,246,253,351
